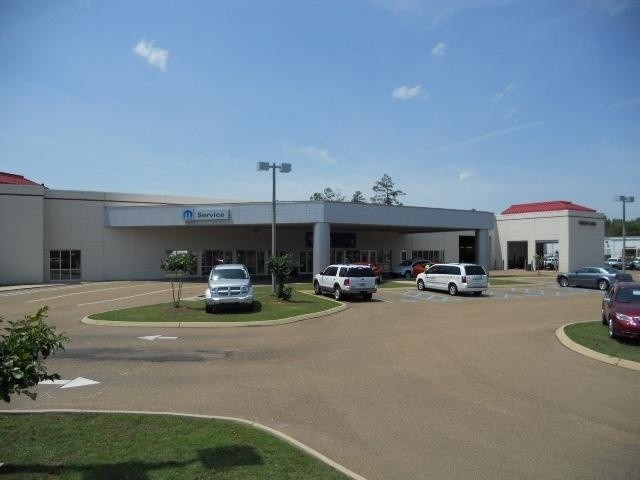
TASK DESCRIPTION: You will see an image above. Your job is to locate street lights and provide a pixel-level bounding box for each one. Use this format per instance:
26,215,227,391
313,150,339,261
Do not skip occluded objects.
257,161,292,292
613,195,634,273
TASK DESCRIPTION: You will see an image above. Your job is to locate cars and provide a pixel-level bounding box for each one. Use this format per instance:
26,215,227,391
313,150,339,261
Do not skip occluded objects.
391,257,487,296
313,261,384,301
204,264,253,313
543,257,640,344
